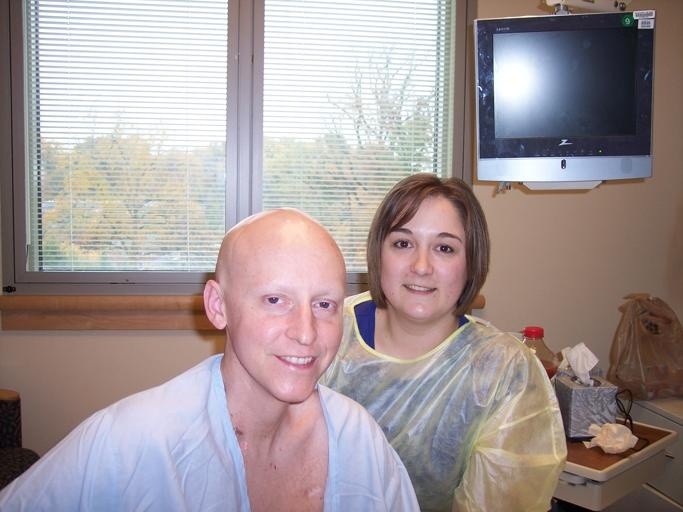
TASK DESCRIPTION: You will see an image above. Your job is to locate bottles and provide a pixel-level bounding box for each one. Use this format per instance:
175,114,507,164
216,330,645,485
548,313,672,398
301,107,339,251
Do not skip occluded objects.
522,326,558,380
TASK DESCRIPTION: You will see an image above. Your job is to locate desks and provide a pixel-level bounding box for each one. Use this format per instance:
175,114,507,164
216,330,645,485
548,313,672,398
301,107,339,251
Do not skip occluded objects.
556,415,676,511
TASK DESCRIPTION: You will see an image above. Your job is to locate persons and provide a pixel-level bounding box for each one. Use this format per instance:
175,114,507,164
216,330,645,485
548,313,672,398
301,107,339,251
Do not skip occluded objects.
315,171,569,512
0,206,423,512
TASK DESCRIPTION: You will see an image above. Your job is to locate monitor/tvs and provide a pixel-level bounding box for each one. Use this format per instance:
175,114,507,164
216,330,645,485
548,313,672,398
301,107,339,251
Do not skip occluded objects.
473,8,655,189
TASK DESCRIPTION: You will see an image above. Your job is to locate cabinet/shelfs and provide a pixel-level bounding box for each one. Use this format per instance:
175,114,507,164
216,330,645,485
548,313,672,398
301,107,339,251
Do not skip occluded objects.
607,389,682,511
